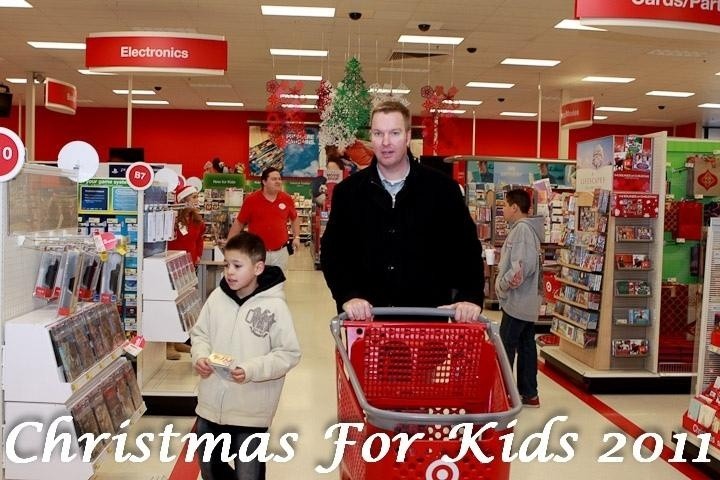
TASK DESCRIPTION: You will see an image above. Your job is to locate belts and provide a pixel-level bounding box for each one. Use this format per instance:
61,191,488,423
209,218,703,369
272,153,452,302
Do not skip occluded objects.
265,241,287,251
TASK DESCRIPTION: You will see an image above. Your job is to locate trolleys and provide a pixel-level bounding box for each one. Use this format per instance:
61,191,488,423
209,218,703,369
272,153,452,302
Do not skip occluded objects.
304,210,313,248
328,303,522,480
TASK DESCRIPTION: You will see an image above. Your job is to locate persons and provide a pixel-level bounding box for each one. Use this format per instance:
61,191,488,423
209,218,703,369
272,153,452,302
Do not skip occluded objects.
319,103,484,322
470,160,494,183
226,167,301,278
494,189,544,408
633,154,650,168
537,162,558,188
189,232,303,479
622,341,629,351
618,255,623,267
164,187,206,360
646,229,650,237
326,144,358,177
626,134,641,152
637,311,645,321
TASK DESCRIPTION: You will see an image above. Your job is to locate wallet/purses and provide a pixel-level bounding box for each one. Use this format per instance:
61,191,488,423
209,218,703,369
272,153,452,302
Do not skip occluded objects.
287,239,294,255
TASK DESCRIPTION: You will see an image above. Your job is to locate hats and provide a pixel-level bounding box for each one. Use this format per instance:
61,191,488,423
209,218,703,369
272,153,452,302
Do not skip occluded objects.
175,186,198,204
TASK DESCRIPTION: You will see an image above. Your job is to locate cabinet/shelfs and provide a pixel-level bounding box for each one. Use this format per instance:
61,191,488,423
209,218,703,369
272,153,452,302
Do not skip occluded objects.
5,298,148,480
193,189,313,243
469,185,661,373
142,249,202,417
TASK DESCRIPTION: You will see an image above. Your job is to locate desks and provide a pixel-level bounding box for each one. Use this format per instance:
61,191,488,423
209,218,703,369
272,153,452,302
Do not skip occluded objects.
197,261,224,304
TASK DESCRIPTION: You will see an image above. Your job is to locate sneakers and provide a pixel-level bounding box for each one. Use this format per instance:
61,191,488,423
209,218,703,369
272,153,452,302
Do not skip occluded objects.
507,395,540,408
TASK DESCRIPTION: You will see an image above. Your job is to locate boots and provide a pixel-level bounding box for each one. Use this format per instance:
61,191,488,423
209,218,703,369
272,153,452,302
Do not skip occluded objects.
167,342,191,360
